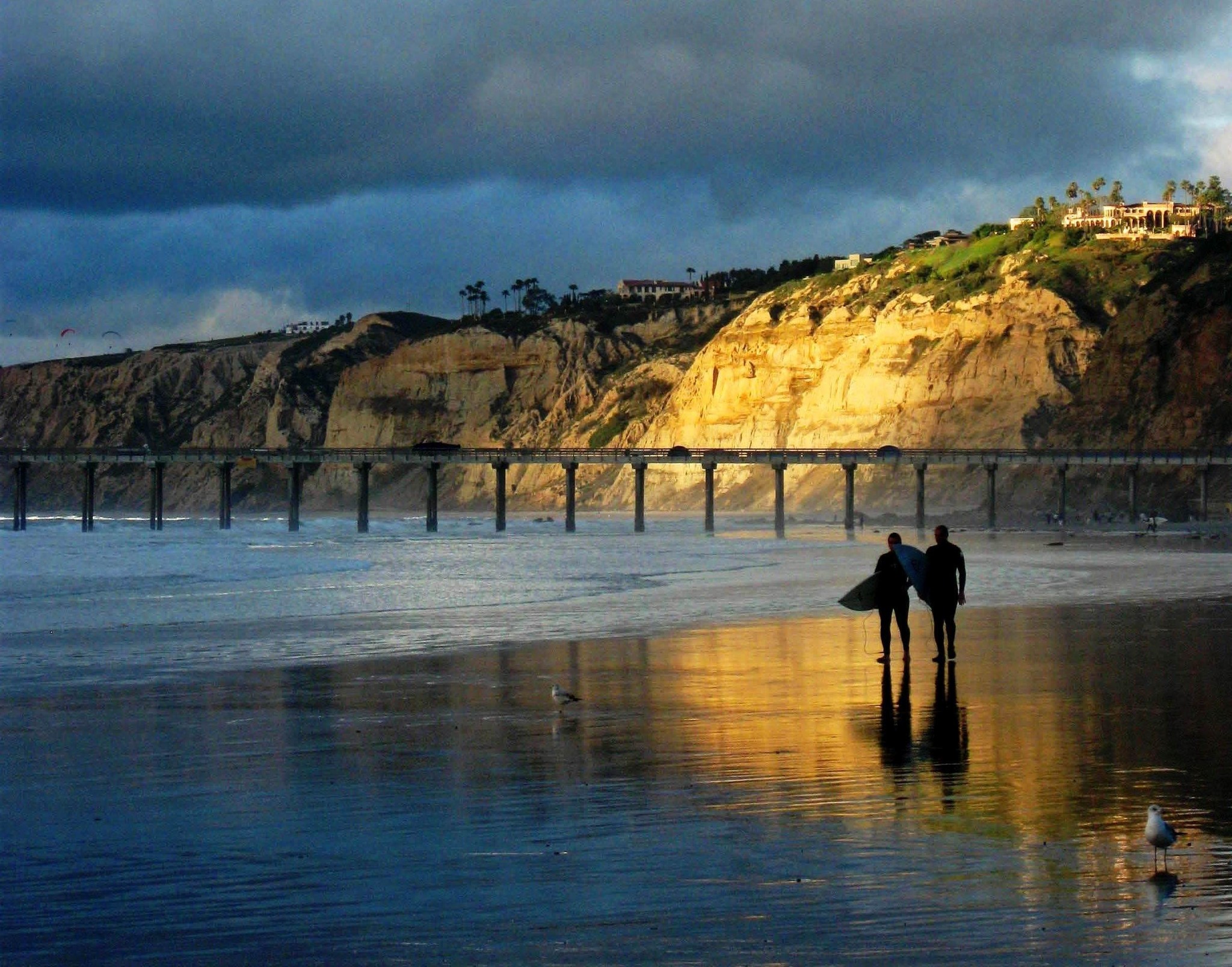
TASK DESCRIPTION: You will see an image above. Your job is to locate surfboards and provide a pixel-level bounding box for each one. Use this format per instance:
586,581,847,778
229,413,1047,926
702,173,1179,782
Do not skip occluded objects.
837,572,882,612
891,545,932,610
1133,517,1169,527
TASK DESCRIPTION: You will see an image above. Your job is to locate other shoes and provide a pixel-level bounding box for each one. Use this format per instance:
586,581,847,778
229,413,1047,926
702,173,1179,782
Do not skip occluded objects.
947,645,956,659
932,655,946,663
903,651,910,660
877,656,891,665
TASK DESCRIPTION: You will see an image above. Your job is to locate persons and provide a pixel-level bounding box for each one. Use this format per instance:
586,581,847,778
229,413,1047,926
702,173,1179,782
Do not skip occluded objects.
875,532,914,663
917,524,966,663
1046,509,1099,528
1143,515,1157,533
859,516,864,528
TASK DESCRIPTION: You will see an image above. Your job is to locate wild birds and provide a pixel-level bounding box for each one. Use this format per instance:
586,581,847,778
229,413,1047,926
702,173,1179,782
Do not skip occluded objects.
548,683,583,712
1145,863,1180,917
1144,805,1187,863
